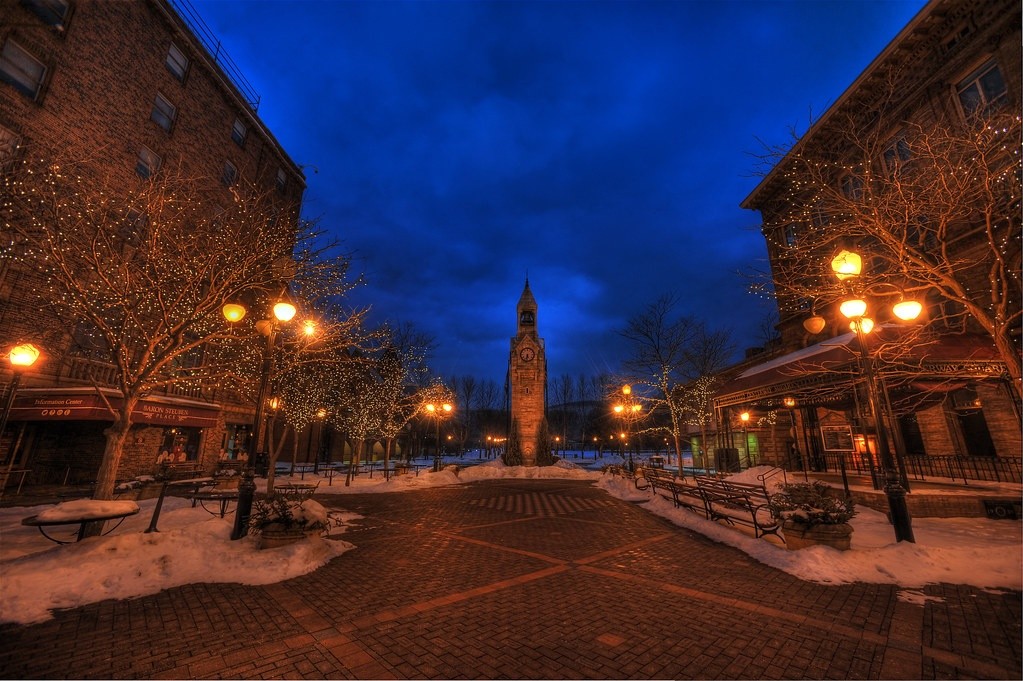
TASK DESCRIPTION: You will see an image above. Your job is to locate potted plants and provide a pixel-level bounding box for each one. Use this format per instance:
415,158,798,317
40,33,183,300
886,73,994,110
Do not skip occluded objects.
768,479,860,552
242,482,343,549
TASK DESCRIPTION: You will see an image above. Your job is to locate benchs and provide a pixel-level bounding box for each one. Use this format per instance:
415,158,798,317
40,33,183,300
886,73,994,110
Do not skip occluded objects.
216,461,248,476
693,476,785,543
611,465,636,480
649,476,676,507
642,467,675,485
162,460,206,479
670,481,708,519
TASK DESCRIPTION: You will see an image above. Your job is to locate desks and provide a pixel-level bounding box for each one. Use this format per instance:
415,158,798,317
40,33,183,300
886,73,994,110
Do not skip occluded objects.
20,506,141,544
345,465,364,481
377,468,401,482
184,491,238,518
180,481,219,508
274,484,318,496
320,467,344,486
294,464,315,480
363,463,381,478
413,465,426,477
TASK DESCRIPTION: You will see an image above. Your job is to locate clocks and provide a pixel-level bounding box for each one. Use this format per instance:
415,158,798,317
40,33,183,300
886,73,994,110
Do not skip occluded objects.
520,347,535,362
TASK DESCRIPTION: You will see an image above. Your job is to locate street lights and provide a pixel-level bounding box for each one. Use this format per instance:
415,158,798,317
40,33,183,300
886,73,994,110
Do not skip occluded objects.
614,384,642,473
609,435,614,456
620,433,626,459
803,247,922,544
741,412,753,468
447,435,452,456
425,377,453,473
663,438,669,455
0,341,41,433
556,437,560,455
488,436,507,459
784,398,803,472
221,282,320,540
594,436,598,461
